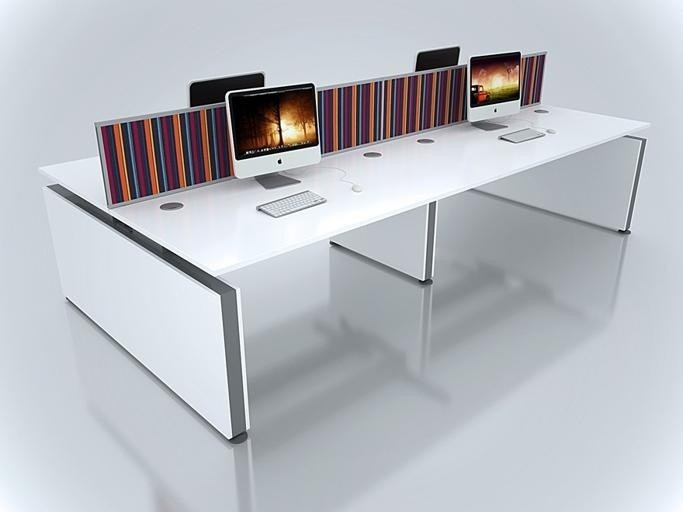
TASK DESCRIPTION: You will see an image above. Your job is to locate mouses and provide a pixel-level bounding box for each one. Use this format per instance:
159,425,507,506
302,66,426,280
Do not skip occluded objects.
352,184,360,192
546,128,555,134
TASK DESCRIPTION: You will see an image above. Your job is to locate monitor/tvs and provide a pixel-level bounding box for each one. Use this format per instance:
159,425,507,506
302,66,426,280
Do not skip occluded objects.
224,81,321,189
190,72,264,107
467,51,521,130
415,46,460,72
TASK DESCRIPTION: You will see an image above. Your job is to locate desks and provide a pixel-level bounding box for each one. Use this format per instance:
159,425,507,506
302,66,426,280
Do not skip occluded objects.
39,101,653,445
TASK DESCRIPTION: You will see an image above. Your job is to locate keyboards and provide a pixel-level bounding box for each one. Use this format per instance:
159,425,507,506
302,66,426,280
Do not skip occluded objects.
498,128,545,143
256,190,326,218
